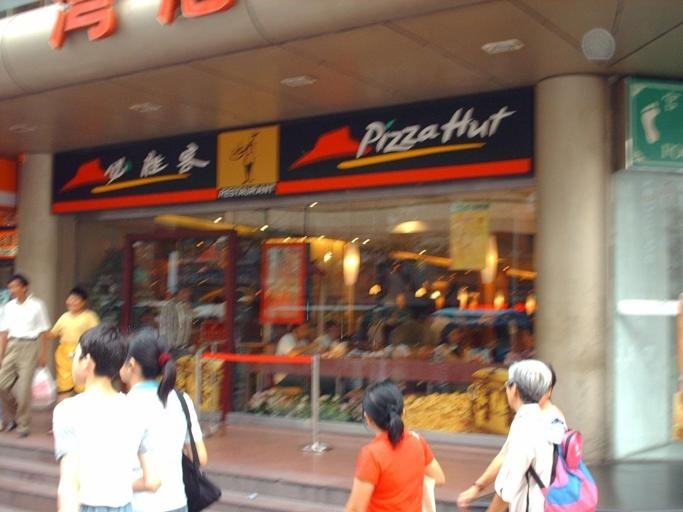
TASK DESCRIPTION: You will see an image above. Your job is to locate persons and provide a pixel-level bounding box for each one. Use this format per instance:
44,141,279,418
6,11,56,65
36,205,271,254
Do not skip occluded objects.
273,319,354,399
456,362,566,512
0,276,52,438
431,323,533,394
354,289,436,395
47,288,102,434
484,358,563,512
344,381,446,512
158,284,262,400
52,322,162,512
119,326,208,512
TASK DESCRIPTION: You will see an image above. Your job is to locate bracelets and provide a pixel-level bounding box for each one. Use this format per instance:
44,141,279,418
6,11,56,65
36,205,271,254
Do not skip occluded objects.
473,483,483,491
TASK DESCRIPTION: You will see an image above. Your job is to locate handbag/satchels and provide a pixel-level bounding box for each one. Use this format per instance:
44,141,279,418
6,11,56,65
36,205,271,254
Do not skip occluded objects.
407,429,437,512
172,387,222,512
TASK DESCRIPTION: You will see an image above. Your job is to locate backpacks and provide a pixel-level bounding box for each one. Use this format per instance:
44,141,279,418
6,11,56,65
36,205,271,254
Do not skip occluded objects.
525,422,600,512
